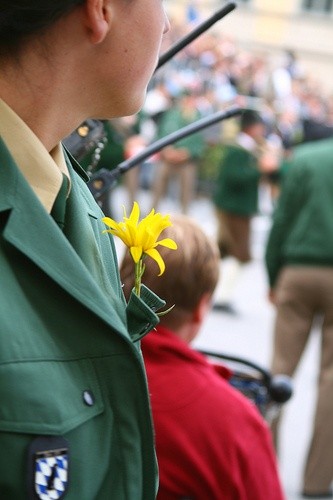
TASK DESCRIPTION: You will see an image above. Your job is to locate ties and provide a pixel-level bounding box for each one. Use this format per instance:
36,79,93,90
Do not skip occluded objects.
48,173,69,236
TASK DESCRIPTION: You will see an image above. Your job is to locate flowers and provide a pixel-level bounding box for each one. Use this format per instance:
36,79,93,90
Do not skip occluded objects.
100,200,179,318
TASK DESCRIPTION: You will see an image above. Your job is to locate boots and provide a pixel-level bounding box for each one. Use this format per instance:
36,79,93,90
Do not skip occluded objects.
230,256,251,317
212,255,234,315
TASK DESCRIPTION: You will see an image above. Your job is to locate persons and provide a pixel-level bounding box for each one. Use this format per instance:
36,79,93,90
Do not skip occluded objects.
214,107,281,322
119,214,283,500
76,88,210,215
265,138,332,499
0,1,167,499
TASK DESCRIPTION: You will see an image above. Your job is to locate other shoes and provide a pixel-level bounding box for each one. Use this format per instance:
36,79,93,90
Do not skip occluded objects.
301,490,333,500
